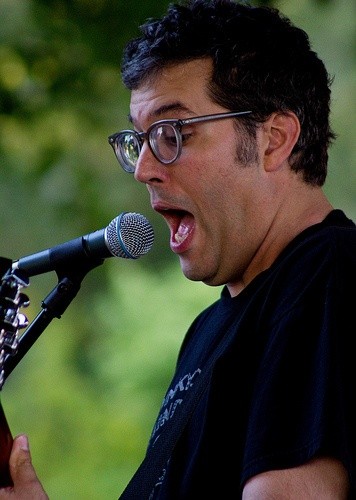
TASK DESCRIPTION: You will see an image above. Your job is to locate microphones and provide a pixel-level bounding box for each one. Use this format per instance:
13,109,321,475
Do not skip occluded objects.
5,211,155,282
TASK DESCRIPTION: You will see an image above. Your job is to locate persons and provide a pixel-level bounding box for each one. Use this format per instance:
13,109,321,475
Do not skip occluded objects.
0,2,356,500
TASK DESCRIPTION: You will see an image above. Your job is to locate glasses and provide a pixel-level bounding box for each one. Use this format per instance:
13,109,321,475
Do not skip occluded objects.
107,110,253,174
